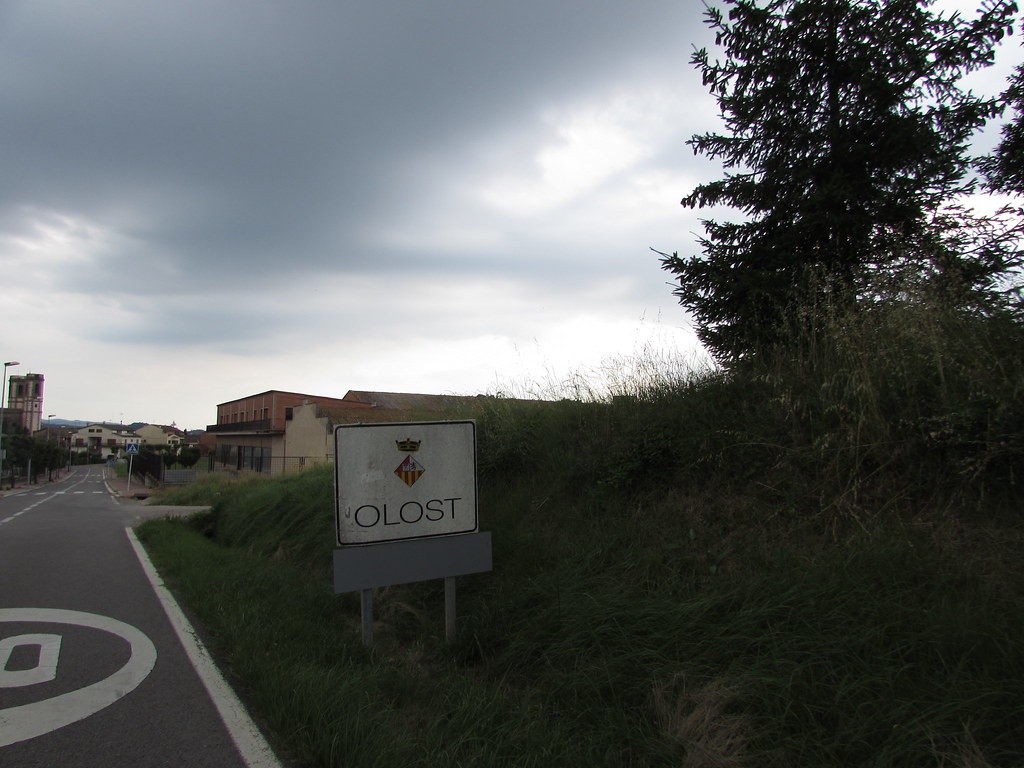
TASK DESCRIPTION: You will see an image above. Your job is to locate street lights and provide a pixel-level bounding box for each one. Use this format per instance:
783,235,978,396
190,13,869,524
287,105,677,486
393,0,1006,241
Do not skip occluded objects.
0,361,20,491
27,397,43,485
57,425,65,447
44,414,56,480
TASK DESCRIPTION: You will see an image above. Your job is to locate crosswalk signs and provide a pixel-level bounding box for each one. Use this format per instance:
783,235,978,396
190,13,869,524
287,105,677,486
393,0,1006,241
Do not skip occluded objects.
126,442,139,455
